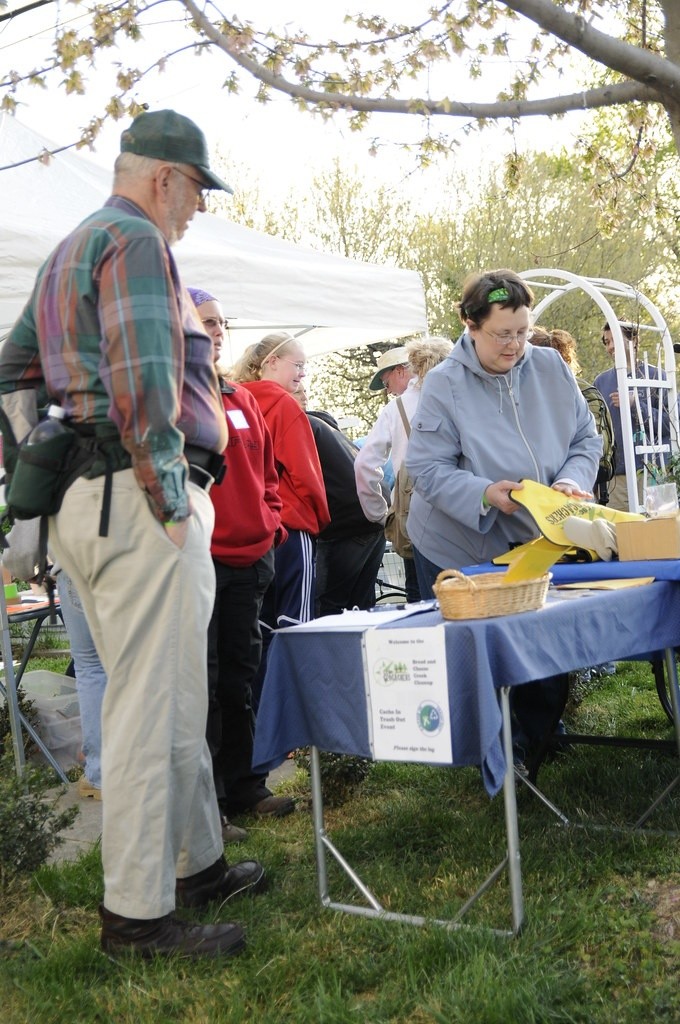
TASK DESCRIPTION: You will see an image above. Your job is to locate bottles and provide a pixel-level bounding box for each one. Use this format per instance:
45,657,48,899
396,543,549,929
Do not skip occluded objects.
28,405,66,447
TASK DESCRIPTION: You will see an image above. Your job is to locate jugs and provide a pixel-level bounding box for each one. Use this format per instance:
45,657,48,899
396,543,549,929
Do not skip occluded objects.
643,483,679,518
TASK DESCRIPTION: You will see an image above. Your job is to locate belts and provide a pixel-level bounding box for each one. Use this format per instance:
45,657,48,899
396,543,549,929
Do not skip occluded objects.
188,466,210,491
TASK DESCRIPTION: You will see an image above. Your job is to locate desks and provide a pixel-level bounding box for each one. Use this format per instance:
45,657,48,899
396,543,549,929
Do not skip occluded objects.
252,577,680,937
0,588,62,795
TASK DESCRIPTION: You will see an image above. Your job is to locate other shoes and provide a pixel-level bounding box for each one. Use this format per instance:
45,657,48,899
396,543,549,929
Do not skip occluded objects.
4,583,22,604
80,778,103,800
219,815,249,842
513,761,529,784
595,662,617,677
238,794,294,821
575,666,592,683
554,719,567,736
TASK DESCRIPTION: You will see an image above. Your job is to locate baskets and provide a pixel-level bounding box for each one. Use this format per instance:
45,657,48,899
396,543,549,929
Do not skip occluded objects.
431,569,553,620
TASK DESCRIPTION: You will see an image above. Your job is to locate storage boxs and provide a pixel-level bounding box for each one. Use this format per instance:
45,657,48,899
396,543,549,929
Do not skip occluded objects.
0,669,85,773
616,516,680,562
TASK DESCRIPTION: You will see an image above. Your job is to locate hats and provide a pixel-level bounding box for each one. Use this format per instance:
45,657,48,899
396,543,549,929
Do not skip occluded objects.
121,110,235,196
368,346,410,391
565,515,617,562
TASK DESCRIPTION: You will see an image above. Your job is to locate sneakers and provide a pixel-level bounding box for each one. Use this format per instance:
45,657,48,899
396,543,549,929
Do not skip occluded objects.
98,901,248,966
176,854,273,912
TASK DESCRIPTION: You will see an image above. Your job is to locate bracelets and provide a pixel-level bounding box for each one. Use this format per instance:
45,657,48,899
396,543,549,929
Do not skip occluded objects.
165,520,178,526
482,495,490,508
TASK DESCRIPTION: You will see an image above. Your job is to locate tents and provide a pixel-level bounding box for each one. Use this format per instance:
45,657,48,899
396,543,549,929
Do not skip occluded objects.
0,115,428,788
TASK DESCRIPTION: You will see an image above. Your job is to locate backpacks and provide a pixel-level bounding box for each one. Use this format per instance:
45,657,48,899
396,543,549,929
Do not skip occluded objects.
384,396,415,559
574,375,617,483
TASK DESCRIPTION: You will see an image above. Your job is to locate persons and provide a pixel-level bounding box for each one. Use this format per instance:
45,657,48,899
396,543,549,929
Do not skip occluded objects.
0,109,268,958
530,320,680,681
405,269,604,796
0,288,454,843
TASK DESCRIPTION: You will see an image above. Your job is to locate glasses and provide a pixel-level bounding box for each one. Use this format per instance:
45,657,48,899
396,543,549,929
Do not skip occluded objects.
174,168,212,203
201,318,228,329
382,367,395,389
478,325,538,345
285,359,306,370
